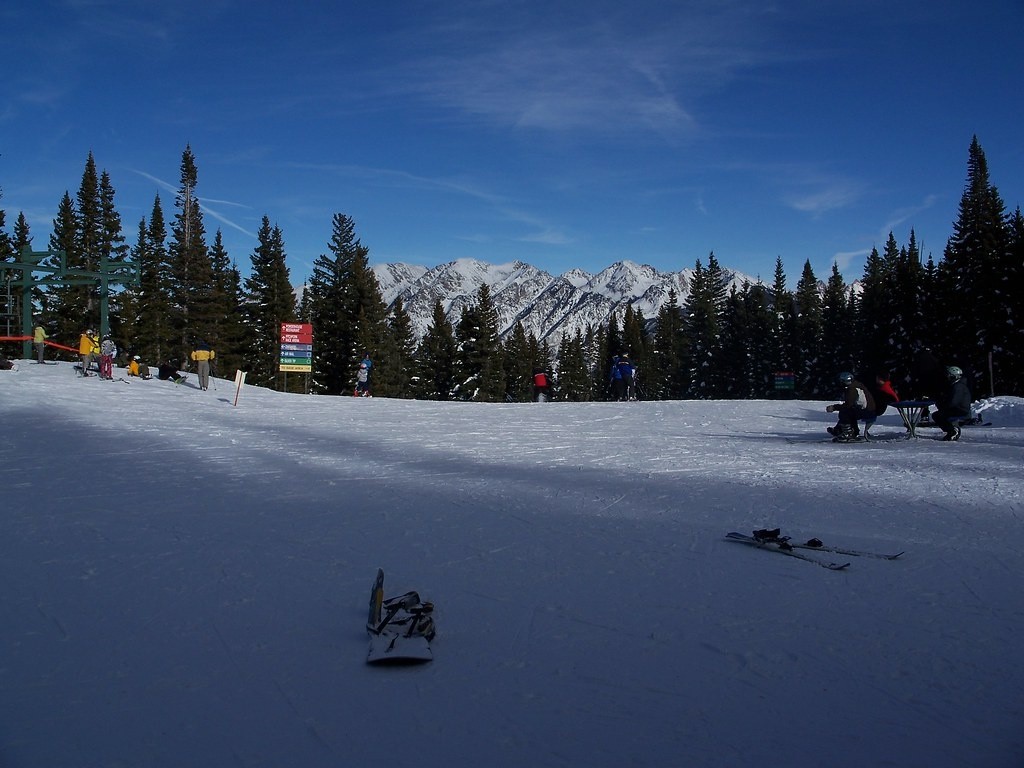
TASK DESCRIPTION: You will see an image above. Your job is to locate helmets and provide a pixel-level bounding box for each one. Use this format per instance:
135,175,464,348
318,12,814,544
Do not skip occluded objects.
133,356,141,360
86,329,94,335
946,366,963,379
622,352,629,358
360,363,367,369
839,372,854,380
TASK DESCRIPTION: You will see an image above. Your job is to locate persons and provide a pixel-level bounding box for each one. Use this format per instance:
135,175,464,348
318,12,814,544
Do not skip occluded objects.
79,329,117,380
611,351,637,402
34,322,49,363
931,366,970,440
531,365,553,402
827,369,899,436
118,351,186,383
827,372,875,443
191,339,215,390
355,354,372,397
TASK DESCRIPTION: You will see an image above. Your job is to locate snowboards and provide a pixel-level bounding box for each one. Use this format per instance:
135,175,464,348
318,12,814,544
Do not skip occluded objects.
362,565,437,666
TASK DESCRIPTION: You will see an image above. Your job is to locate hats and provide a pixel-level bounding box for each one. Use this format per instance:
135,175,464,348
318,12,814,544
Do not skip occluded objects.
42,323,48,329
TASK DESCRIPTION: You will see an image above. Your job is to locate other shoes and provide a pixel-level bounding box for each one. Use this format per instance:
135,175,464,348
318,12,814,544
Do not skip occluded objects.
175,377,187,384
84,373,88,377
919,416,929,423
100,377,113,380
628,397,638,402
201,387,207,391
362,390,371,397
354,390,359,397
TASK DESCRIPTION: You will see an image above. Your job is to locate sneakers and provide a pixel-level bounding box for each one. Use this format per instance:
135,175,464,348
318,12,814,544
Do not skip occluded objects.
827,425,842,436
944,426,961,441
832,423,856,442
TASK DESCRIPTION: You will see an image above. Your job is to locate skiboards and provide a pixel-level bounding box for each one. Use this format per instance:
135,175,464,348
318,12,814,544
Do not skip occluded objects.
723,530,905,571
